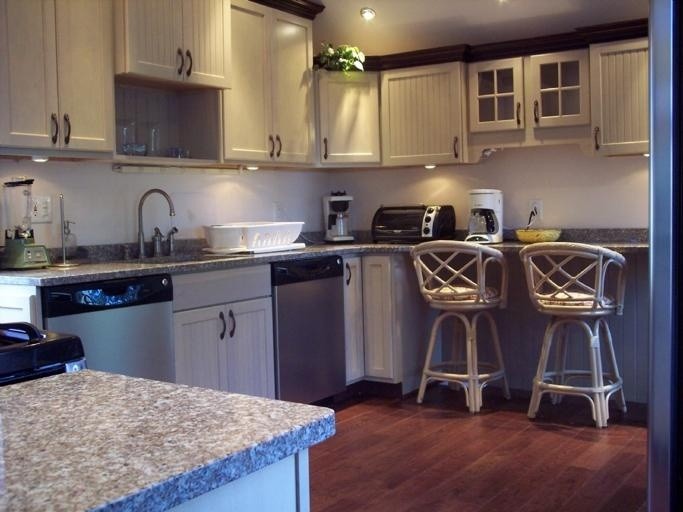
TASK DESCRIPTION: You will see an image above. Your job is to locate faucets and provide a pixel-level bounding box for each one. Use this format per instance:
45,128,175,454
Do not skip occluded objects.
168,226,178,255
136,187,176,259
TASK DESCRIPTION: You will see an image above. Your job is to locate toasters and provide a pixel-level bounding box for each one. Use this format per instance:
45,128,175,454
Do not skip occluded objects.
368,199,458,247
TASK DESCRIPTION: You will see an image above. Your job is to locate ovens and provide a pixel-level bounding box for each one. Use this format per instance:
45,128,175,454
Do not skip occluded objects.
31,270,177,396
268,254,350,411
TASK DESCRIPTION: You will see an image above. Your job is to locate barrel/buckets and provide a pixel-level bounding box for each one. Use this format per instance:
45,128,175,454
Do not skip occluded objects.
123,253,250,265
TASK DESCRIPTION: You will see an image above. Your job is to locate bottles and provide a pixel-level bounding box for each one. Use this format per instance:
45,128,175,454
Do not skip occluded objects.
117,119,163,156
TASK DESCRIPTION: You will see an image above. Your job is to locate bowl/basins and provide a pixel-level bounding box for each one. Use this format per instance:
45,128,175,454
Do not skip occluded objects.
513,225,564,245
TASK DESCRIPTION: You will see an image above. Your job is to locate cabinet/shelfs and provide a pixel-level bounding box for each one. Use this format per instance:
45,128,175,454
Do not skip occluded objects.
358,255,447,398
315,53,380,167
338,246,365,396
0,0,116,159
114,2,236,93
170,260,275,401
457,33,592,163
172,1,322,168
379,44,466,167
576,17,649,160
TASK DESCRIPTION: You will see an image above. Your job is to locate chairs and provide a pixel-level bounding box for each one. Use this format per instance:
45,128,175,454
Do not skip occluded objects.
516,238,633,430
409,238,510,412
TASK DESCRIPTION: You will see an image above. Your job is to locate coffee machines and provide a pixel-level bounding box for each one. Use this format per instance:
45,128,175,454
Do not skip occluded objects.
319,187,357,243
462,187,507,245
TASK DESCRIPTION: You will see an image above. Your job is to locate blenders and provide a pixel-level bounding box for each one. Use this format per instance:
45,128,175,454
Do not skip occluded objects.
1,170,53,271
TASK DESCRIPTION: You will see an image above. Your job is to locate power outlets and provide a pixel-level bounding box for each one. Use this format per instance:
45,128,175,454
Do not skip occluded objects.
528,199,545,226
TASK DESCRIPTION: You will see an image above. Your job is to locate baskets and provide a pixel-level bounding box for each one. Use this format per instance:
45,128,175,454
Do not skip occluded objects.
197,218,307,251
